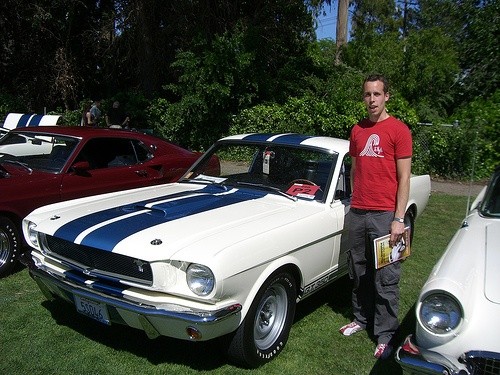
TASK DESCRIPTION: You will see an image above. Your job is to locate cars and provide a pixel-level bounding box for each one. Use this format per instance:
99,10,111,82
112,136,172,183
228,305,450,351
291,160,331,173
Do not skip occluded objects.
0,125,221,277
394,169,499,375
21,133,432,369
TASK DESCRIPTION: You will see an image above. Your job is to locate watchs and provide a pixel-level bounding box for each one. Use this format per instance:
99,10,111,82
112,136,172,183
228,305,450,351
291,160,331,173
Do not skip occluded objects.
393,216,404,222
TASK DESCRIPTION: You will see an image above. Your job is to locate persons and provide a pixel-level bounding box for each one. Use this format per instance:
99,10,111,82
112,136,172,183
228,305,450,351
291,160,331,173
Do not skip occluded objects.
105,101,129,129
338,74,413,361
80,97,105,128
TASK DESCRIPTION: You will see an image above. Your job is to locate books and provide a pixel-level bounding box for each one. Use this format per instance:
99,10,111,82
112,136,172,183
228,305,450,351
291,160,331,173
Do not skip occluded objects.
374,224,411,268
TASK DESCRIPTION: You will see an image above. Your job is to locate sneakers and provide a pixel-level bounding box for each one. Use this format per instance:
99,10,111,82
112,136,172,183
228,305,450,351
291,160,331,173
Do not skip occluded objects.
374,343,392,359
339,322,362,335
